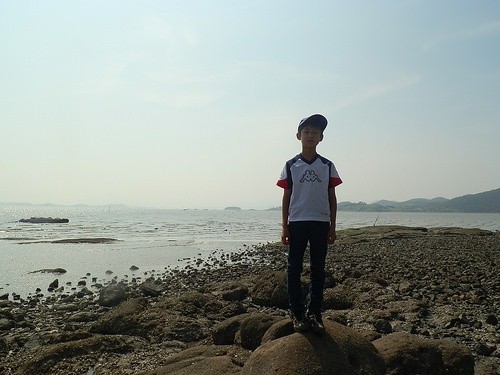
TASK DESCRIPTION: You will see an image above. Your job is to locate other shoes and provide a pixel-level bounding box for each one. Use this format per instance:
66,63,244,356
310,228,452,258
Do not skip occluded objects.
307,312,326,332
291,313,309,331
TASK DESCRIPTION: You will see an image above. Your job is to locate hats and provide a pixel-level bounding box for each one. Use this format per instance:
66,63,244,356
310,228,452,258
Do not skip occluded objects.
298,114,328,133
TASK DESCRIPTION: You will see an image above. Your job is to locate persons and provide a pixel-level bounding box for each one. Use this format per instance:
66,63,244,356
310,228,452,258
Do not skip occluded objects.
275,114,343,334
